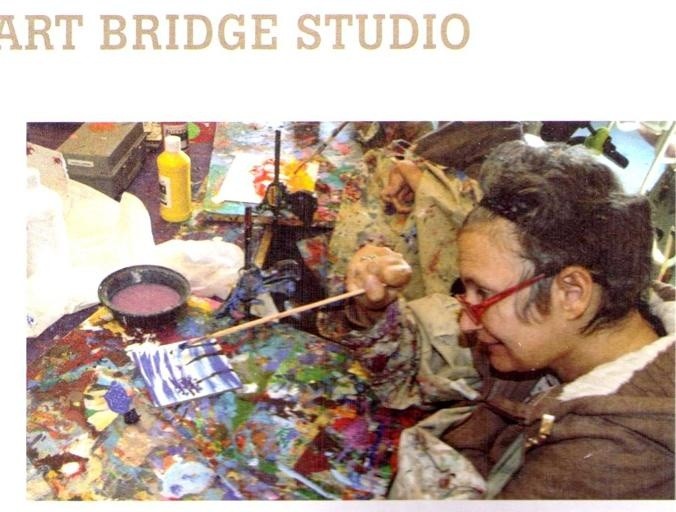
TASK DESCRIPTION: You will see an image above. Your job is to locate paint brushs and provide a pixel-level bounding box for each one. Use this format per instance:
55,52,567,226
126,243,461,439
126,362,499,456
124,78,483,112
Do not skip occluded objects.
178,281,389,350
309,121,349,161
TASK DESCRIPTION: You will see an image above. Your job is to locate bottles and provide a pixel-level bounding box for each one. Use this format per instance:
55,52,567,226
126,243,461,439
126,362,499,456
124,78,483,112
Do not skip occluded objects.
156,135,193,223
161,122,190,156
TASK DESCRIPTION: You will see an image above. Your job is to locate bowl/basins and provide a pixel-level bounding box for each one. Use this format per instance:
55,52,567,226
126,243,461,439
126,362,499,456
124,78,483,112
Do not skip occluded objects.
96,263,192,329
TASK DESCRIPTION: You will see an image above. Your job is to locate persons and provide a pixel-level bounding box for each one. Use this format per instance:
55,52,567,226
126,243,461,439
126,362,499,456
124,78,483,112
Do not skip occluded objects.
344,140,676,500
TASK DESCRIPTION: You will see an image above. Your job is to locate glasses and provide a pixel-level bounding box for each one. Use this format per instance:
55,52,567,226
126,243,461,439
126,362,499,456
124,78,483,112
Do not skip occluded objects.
451,275,553,326
